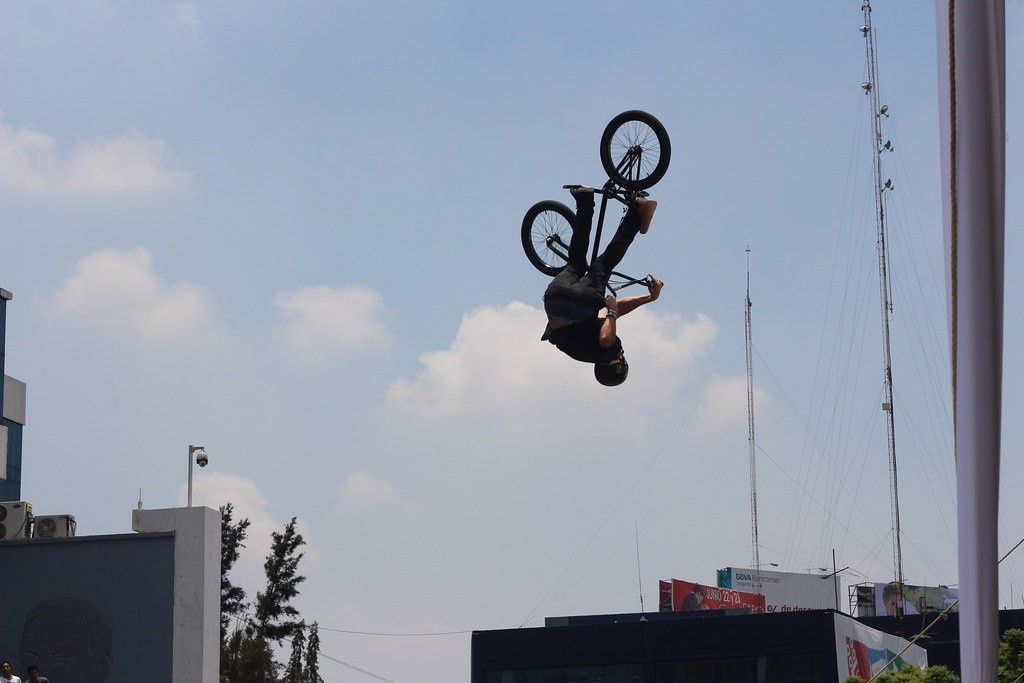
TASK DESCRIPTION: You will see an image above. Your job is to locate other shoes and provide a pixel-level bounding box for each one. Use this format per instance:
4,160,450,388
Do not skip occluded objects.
569,188,595,210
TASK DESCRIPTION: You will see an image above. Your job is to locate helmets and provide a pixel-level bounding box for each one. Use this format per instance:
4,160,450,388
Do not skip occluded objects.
595,362,629,387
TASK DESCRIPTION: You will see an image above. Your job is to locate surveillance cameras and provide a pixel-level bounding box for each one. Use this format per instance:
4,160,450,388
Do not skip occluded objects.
196,451,208,467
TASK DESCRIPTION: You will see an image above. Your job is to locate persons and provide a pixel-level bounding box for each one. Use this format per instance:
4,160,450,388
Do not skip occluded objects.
681,584,710,611
541,187,662,387
0,661,49,683
878,585,900,616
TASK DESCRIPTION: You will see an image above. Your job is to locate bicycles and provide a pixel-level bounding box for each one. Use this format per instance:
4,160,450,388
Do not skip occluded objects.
521,110,672,308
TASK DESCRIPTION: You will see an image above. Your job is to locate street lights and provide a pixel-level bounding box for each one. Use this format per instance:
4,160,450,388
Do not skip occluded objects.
187,444,209,507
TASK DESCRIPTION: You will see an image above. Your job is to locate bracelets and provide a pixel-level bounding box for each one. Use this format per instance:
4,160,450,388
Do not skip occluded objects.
607,309,618,319
